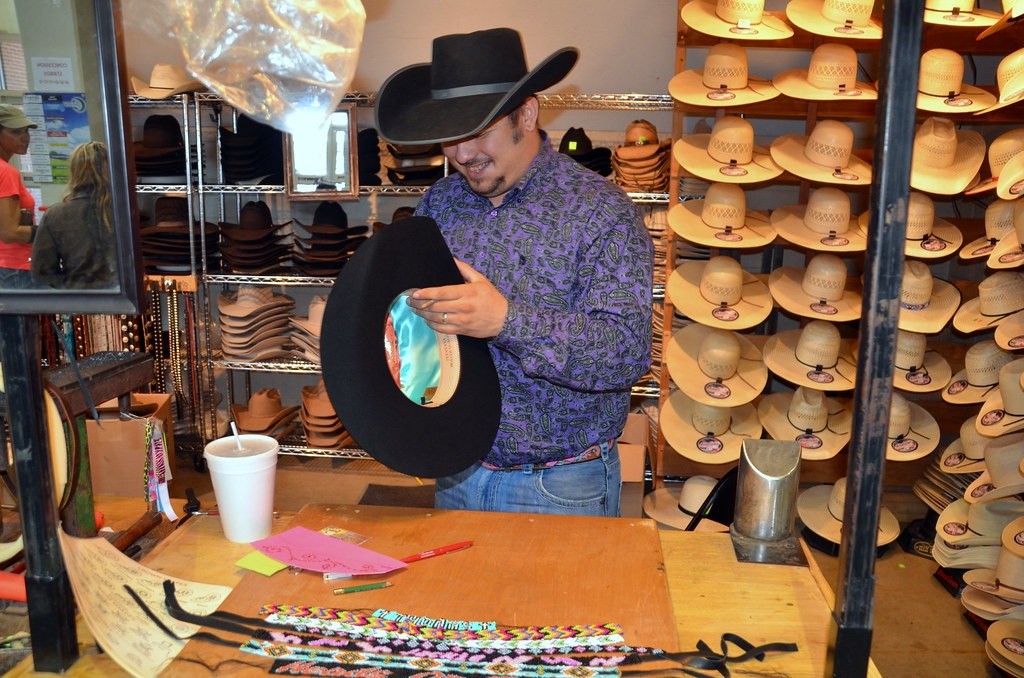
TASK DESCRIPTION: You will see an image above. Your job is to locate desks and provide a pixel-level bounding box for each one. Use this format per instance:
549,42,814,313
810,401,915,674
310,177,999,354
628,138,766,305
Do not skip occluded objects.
0,502,885,678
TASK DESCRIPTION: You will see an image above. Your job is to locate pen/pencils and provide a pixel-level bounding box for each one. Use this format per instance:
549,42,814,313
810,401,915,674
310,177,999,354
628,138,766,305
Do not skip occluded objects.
400,541,474,563
333,581,393,595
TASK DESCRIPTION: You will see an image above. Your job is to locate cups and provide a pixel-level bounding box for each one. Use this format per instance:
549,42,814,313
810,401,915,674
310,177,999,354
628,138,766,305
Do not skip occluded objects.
202,433,280,543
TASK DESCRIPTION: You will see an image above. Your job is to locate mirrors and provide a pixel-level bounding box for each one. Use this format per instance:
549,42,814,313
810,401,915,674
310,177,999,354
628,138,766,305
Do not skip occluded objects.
0,0,144,315
284,101,359,201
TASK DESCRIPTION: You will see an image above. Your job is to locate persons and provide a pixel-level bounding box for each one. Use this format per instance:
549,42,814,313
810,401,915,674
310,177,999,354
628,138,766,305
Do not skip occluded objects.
375,28,655,516
0,104,38,289
30,141,119,290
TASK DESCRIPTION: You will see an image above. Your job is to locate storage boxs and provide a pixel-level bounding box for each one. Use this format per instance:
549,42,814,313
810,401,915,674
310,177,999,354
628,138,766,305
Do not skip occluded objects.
86,392,176,495
615,413,655,516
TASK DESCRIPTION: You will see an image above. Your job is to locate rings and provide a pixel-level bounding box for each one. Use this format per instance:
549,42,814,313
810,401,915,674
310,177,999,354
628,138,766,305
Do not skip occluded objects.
443,312,447,324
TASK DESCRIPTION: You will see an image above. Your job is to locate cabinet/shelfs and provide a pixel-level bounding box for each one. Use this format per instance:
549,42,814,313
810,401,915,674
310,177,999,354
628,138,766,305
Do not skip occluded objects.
119,0,1024,544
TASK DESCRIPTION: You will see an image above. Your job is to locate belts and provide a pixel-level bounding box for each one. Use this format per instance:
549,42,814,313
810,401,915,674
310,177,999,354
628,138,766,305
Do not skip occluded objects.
480,441,615,469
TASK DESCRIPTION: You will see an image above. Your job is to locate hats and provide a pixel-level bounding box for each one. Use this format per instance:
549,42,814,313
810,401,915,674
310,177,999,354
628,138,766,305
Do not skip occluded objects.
214,283,331,366
139,198,413,276
0,103,34,128
217,114,287,185
319,216,501,478
357,125,449,186
131,62,208,99
134,114,207,176
231,380,357,450
960,584,1024,678
635,0,1022,598
556,118,671,195
375,27,581,145
